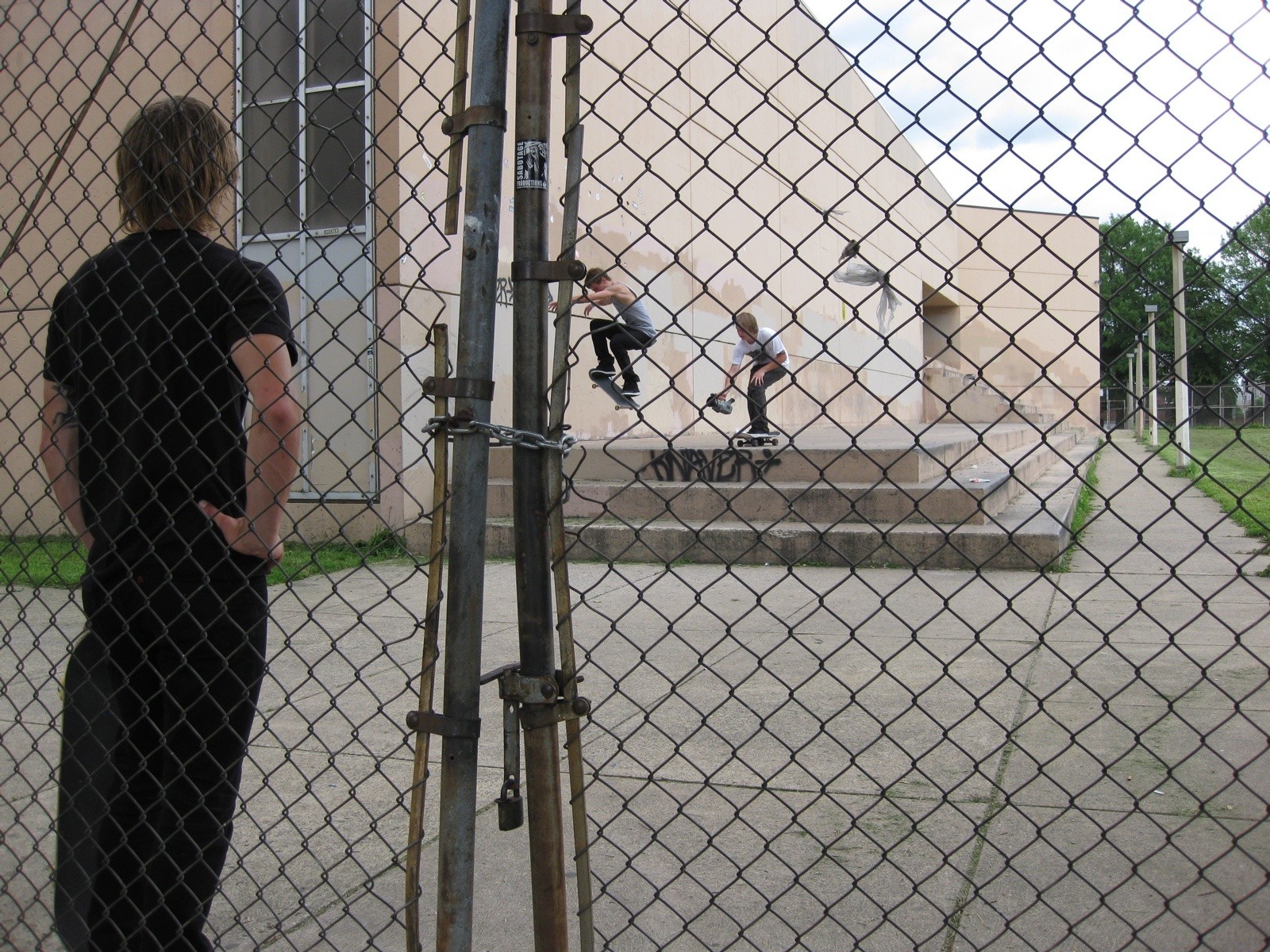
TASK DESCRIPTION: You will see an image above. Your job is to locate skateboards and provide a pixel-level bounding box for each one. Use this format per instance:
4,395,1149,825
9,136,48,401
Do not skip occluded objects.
589,370,639,411
732,431,782,447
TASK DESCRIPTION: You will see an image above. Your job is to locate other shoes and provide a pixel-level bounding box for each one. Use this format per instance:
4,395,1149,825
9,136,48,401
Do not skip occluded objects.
748,427,769,437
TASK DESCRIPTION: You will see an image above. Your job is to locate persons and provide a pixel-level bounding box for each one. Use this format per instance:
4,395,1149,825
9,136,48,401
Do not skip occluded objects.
716,312,790,434
37,95,302,952
547,267,658,396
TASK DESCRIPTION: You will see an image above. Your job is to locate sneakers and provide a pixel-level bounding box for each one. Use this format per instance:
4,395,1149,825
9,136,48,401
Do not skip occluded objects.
621,380,641,396
590,363,616,378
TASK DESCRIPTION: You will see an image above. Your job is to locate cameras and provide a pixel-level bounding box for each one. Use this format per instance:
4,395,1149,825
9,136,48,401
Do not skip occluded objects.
706,393,735,415
